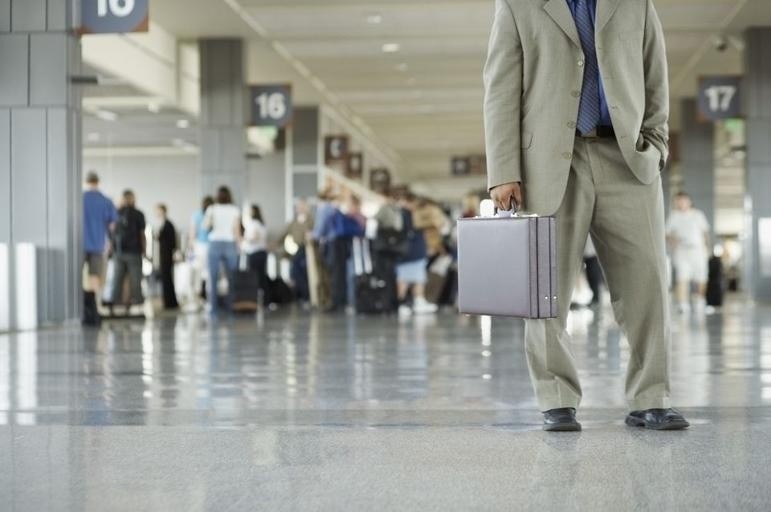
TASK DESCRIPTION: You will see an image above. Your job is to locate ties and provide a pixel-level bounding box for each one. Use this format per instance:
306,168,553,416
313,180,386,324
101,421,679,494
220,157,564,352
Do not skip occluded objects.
574,1,598,136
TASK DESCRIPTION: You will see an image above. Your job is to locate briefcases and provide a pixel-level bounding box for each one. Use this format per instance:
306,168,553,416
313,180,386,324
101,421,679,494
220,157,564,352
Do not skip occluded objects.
458,200,558,316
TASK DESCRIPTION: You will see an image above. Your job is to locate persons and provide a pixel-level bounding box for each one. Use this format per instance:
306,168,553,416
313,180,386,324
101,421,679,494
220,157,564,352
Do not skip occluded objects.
80,170,715,325
479,0,694,435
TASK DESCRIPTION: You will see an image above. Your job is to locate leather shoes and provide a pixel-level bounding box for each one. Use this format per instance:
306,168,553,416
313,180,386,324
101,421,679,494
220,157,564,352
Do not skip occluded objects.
625,408,688,430
542,408,581,430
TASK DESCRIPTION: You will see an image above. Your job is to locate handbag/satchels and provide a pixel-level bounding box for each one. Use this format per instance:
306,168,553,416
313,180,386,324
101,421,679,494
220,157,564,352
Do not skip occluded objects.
331,210,362,237
372,230,407,260
706,257,725,306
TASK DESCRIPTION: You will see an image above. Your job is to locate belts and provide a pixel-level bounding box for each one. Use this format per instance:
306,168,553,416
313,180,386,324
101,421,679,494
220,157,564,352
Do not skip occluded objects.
574,125,615,138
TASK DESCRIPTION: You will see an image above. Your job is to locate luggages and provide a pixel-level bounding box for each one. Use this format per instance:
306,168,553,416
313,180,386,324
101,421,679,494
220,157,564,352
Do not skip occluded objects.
424,248,455,307
365,271,397,312
352,236,368,314
